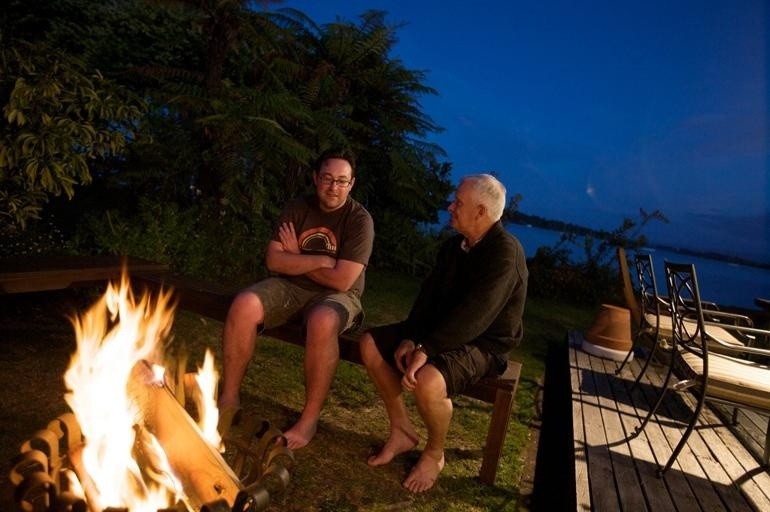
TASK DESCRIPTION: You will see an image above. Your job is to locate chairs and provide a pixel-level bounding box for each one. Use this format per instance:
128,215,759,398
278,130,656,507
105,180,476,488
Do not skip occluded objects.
614,246,754,427
629,257,765,479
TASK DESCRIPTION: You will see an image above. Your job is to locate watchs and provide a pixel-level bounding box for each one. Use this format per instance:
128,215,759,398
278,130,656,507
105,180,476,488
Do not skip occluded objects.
415,342,427,355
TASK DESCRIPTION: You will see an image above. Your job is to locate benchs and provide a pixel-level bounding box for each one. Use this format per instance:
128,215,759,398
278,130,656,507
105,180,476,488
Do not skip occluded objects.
1,253,170,293
136,269,524,487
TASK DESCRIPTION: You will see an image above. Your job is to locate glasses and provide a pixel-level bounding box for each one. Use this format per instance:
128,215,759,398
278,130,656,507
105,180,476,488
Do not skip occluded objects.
315,169,354,188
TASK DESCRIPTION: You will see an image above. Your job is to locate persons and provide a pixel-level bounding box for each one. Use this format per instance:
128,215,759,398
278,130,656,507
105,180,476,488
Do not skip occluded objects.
358,173,529,494
220,147,375,450
